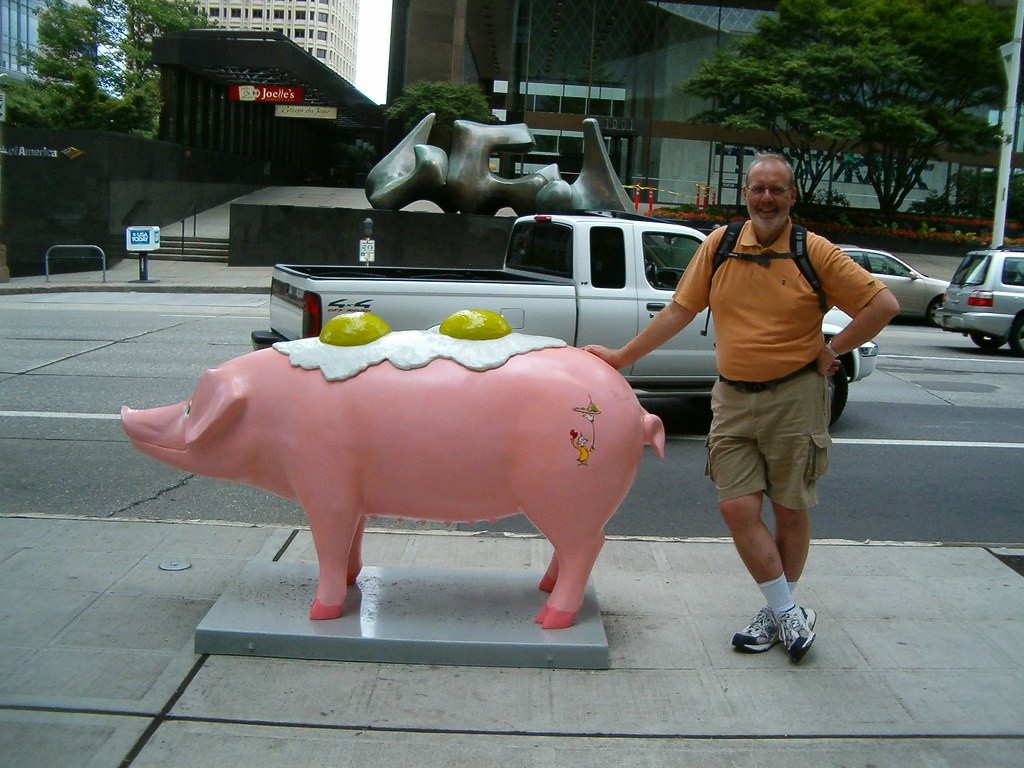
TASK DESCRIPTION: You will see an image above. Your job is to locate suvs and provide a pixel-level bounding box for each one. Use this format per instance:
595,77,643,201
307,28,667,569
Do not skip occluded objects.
941,246,1024,352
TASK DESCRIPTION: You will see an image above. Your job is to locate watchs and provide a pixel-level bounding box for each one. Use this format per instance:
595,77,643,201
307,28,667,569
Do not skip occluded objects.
825,337,839,359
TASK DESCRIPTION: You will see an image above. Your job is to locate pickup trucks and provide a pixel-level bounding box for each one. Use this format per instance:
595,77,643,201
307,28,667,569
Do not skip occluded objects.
251,215,879,428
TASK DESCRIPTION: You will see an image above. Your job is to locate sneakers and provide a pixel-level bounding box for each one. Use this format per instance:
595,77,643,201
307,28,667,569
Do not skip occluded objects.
770,606,817,663
731,605,779,652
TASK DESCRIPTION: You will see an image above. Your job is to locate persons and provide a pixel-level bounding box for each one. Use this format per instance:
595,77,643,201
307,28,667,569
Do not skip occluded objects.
582,152,900,664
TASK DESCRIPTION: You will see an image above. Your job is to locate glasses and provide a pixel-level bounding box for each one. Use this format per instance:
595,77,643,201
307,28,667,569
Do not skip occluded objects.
747,186,791,195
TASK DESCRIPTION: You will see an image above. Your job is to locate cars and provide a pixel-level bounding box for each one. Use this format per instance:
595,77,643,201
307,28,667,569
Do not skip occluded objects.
831,244,951,327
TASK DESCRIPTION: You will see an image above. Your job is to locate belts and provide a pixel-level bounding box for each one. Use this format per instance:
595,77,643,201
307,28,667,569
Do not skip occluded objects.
719,359,817,393
